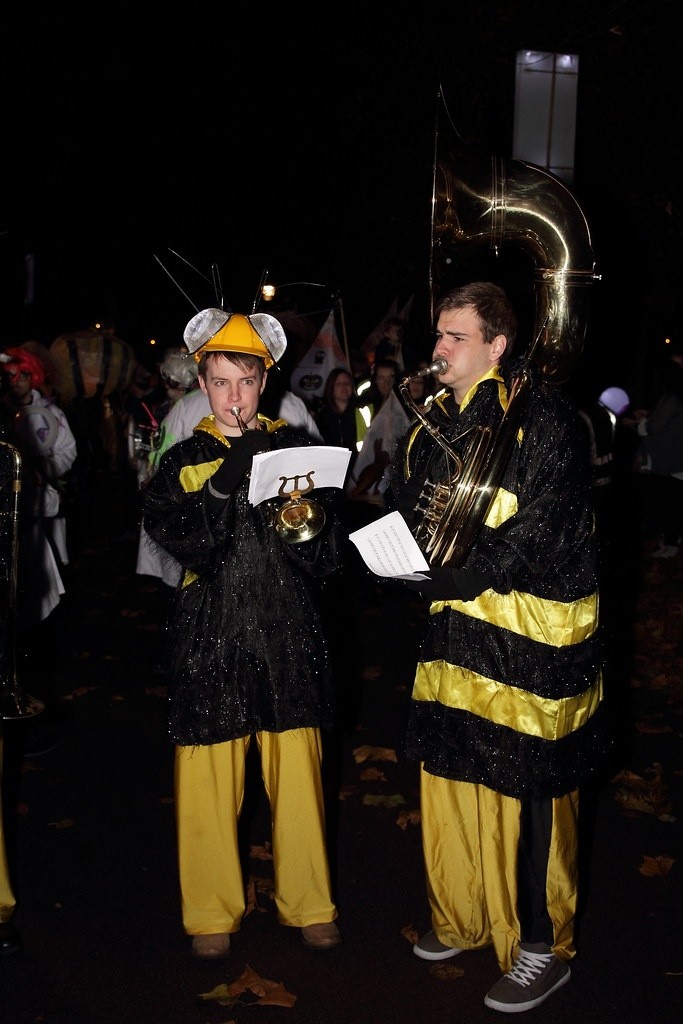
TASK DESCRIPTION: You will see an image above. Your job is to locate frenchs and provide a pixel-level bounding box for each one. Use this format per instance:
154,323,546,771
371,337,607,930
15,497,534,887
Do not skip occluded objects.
389,77,595,608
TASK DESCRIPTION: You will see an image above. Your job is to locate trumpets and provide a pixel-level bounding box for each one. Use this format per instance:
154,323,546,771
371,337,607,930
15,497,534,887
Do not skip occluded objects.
232,406,327,544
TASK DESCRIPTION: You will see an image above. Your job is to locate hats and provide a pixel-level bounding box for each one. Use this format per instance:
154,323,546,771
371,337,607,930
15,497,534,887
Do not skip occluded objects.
185,307,288,370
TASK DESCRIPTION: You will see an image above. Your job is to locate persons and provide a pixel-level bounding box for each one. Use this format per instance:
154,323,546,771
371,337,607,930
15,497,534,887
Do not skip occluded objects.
382,277,609,1016
126,304,353,958
1,347,77,622
573,337,683,481
50,319,447,589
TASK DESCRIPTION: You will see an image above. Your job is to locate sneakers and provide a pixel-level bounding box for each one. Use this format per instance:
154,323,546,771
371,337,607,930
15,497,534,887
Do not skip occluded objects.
484,942,572,1013
415,930,464,961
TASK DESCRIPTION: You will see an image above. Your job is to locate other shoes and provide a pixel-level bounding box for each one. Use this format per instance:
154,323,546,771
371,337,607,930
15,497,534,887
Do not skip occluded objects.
21,736,58,756
651,541,678,557
0,922,20,955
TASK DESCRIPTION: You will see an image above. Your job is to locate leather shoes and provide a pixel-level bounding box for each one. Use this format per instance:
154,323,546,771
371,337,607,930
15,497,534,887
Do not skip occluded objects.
190,934,230,960
301,922,341,948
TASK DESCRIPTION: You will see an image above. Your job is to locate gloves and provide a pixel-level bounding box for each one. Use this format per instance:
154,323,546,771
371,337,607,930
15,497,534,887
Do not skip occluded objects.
211,431,267,493
401,567,465,602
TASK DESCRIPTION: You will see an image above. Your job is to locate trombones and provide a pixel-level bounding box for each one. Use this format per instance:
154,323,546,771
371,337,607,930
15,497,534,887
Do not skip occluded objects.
0,437,45,720
